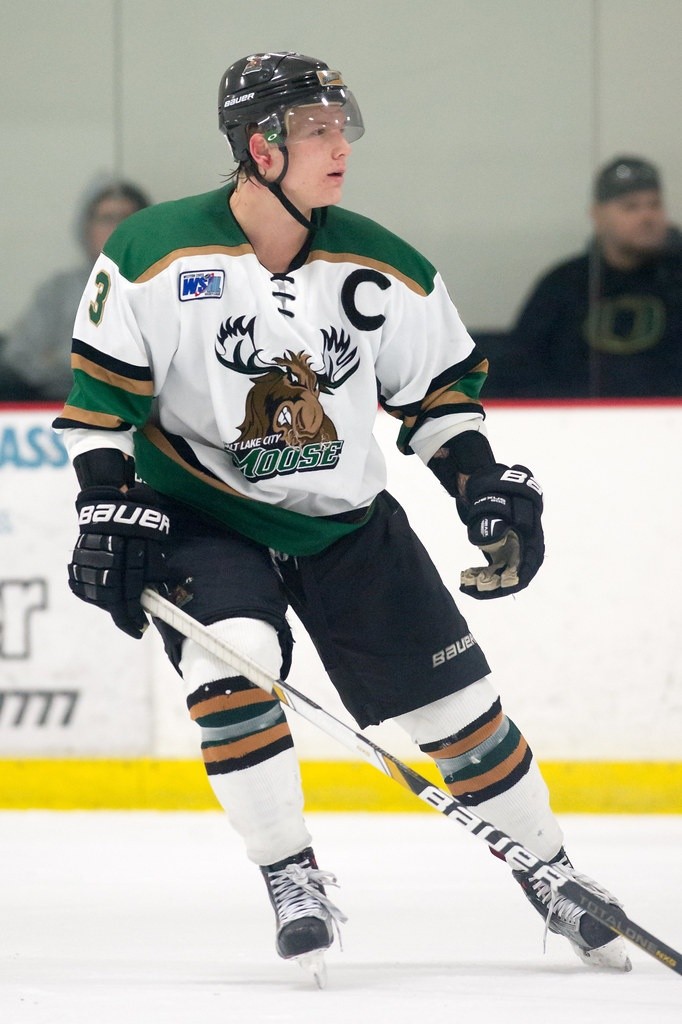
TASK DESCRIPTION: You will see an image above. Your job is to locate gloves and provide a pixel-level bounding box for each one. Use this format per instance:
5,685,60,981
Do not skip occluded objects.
458,462,546,599
67,486,184,638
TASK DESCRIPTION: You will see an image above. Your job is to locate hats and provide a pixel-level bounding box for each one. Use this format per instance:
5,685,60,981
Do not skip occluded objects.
594,159,657,203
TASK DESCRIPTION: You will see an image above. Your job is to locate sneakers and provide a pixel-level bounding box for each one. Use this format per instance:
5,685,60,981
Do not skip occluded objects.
510,847,635,972
260,845,347,993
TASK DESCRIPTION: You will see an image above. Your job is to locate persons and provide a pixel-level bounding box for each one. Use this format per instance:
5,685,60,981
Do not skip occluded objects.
498,157,682,397
4,183,150,399
52,52,632,988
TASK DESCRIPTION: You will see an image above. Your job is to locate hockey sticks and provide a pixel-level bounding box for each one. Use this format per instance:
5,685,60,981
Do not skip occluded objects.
140,583,681,976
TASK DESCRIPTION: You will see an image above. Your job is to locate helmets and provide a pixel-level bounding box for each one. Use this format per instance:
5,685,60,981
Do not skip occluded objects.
216,51,348,163
